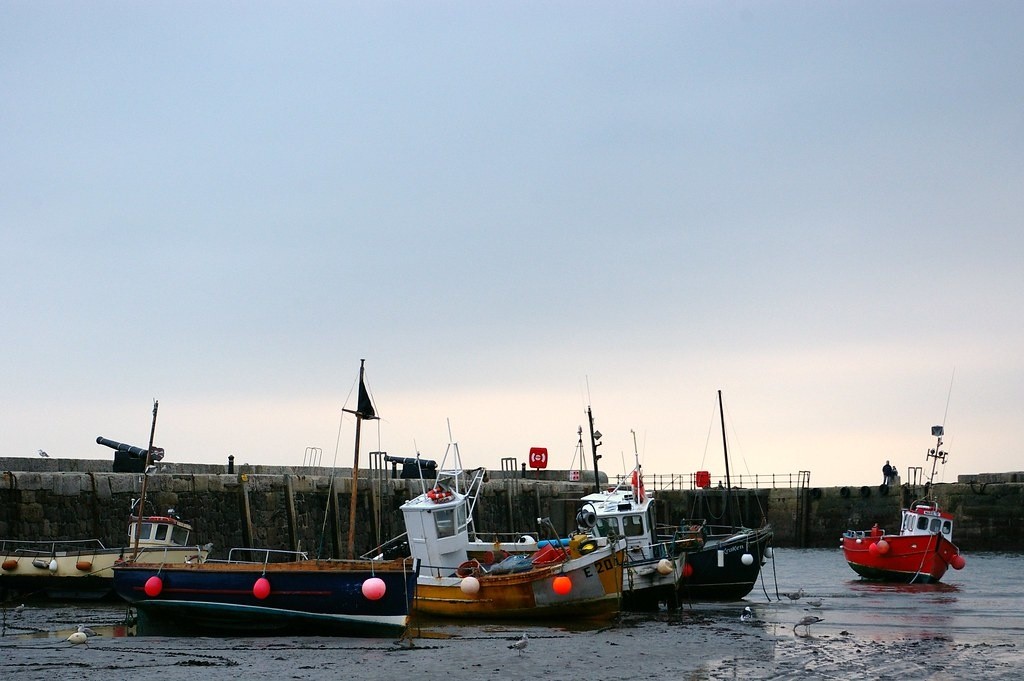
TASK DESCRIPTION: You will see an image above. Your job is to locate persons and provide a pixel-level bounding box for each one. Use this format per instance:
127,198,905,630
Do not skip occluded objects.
882,460,898,485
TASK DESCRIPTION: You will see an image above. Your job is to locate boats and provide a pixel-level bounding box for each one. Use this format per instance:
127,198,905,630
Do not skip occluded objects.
839,424,966,582
109,389,776,639
0,492,213,602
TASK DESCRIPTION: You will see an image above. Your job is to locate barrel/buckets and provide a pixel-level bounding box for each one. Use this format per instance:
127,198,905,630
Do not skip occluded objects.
455,561,480,578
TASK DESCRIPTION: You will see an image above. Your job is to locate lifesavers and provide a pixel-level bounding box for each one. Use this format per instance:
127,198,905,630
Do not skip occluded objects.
427,488,451,499
